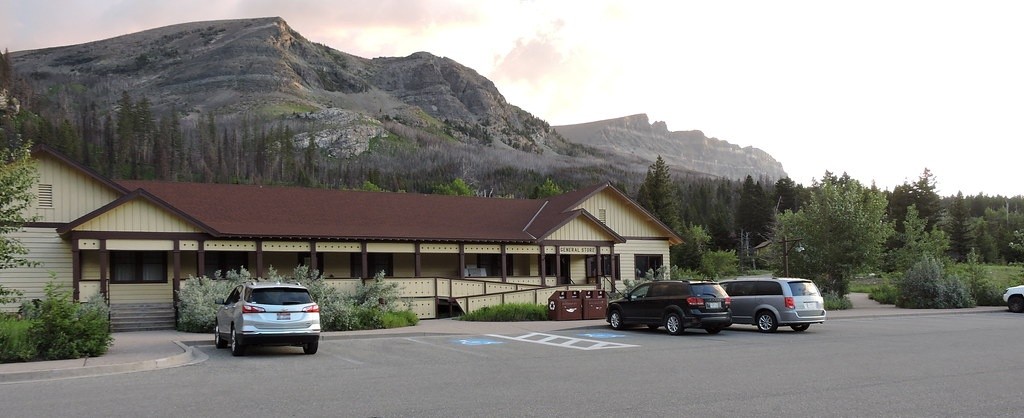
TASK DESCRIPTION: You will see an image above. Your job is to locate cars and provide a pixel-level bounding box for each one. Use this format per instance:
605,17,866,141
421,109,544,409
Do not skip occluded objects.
1002,285,1024,313
606,277,826,335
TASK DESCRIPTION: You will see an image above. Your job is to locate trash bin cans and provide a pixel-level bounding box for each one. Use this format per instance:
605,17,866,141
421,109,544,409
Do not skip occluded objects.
548,290,607,321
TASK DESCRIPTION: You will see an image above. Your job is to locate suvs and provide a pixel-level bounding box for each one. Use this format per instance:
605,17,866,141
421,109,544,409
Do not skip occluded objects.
215,280,321,356
